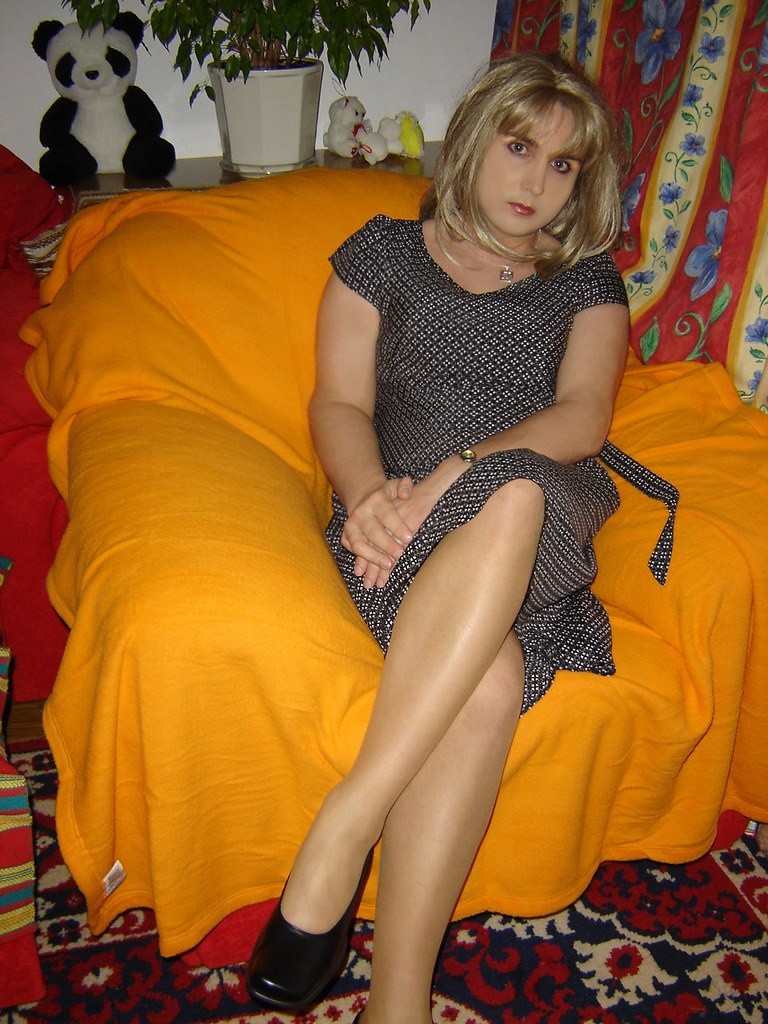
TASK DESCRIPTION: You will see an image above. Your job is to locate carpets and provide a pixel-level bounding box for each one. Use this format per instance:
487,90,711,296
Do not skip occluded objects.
0,748,768,1024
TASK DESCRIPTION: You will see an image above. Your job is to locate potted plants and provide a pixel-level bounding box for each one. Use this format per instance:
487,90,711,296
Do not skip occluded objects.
56,0,432,179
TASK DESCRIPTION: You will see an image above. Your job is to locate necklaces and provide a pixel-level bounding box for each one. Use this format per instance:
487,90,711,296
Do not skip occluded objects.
499,229,541,281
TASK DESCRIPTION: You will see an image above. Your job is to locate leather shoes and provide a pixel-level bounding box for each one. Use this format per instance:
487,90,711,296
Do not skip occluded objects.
246,846,373,1009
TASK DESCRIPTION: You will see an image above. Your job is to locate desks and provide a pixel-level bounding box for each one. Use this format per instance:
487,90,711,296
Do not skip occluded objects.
62,140,449,213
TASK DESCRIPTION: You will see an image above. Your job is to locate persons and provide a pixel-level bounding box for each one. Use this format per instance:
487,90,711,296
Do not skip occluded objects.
244,50,679,1024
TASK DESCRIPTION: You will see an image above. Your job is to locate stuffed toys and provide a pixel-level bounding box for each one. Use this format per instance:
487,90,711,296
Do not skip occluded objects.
356,112,424,165
323,96,372,158
31,11,174,188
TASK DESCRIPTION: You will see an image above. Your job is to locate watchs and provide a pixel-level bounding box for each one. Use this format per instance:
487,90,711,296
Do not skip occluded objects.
458,449,477,465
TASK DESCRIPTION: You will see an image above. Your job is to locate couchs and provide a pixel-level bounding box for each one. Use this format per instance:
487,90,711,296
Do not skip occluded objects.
0,146,768,957
